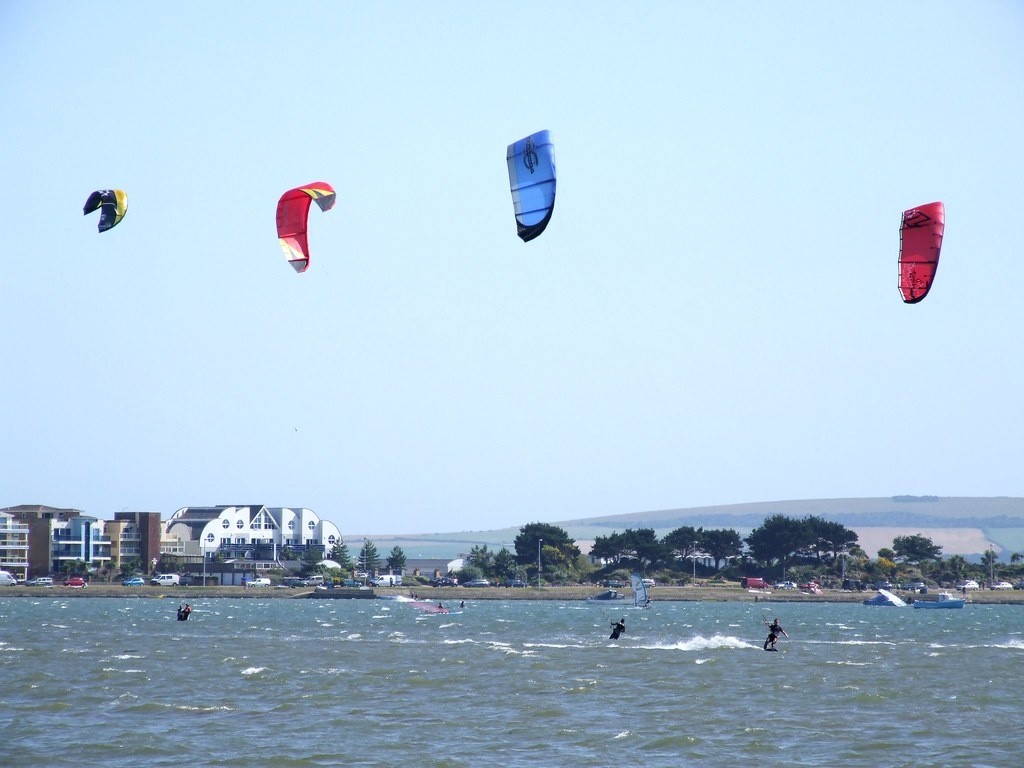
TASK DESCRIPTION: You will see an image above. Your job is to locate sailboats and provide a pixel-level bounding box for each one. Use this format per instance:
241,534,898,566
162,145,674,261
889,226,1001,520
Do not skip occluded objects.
627,572,655,610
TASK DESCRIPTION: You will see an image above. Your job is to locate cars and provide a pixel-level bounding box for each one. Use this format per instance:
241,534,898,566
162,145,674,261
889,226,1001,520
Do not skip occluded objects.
989,582,1013,590
604,579,625,589
461,579,489,587
1012,582,1024,590
955,580,980,592
63,578,85,587
503,579,527,588
741,577,929,590
340,579,362,588
428,577,457,588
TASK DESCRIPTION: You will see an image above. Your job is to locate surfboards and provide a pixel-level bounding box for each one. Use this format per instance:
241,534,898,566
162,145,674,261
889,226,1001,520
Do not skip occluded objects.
765,647,779,652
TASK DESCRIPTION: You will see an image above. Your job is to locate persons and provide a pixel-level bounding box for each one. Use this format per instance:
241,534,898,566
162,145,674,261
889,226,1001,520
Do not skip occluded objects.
411,591,418,600
645,596,651,608
609,618,625,639
764,618,789,651
177,602,192,621
460,600,464,608
438,602,443,608
962,586,966,596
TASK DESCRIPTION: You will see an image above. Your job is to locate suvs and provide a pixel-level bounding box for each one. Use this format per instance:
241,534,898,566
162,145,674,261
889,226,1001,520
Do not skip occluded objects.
247,578,271,587
24,577,53,586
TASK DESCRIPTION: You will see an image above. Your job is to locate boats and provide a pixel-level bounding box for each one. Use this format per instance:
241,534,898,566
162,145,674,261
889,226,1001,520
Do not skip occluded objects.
913,591,967,610
586,589,632,605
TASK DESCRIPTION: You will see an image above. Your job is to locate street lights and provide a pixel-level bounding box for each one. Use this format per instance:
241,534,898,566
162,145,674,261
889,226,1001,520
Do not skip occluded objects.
203,539,207,586
990,543,994,584
538,539,543,587
692,540,698,583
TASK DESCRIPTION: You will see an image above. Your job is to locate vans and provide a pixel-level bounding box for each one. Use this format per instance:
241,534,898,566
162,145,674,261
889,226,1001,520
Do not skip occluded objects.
0,570,17,587
630,578,656,589
367,575,397,588
300,575,324,587
121,574,194,587
